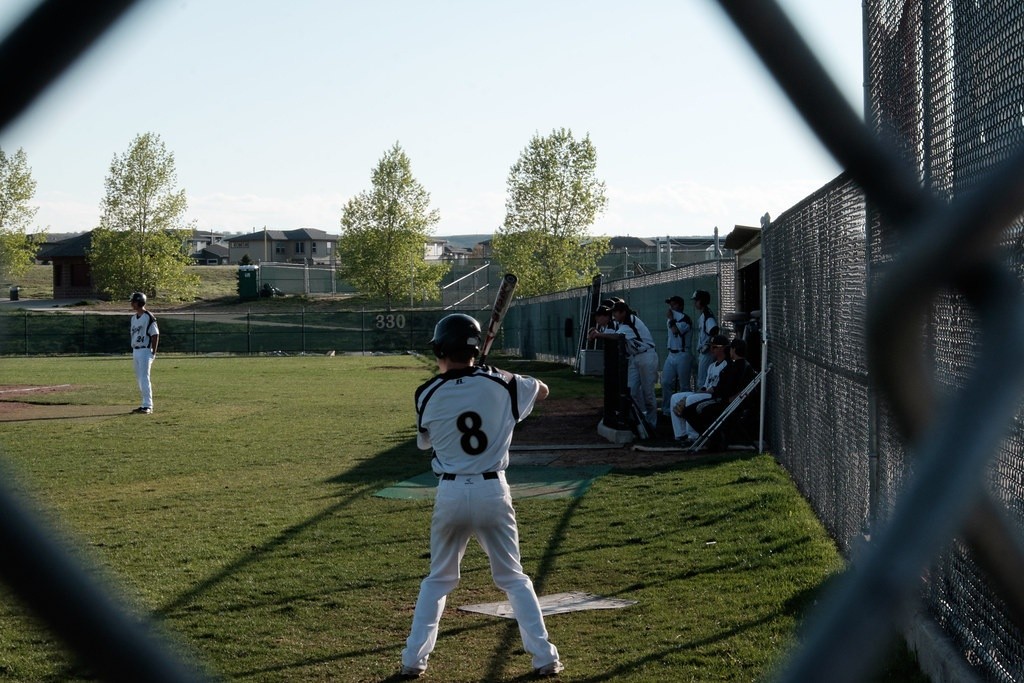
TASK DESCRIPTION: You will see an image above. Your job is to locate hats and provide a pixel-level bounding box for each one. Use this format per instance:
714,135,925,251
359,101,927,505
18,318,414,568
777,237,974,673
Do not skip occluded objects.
591,296,627,316
705,335,729,347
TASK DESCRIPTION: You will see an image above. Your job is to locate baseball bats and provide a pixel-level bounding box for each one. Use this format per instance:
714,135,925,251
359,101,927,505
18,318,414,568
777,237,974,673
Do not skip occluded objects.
630,398,657,442
479,272,519,368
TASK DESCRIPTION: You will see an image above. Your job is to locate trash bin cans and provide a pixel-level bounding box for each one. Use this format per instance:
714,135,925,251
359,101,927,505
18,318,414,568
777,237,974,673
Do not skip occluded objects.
238,269,260,301
9,287,20,300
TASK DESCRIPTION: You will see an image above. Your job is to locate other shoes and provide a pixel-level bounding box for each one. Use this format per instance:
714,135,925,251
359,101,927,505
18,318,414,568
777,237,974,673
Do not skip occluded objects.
133,407,145,412
400,666,425,678
143,409,153,415
681,439,693,448
690,442,722,453
536,660,565,676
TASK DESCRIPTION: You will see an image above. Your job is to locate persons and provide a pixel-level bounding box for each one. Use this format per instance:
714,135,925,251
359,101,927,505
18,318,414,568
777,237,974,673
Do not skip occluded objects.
126,292,159,413
691,290,719,387
587,297,659,427
402,313,565,676
683,339,754,452
657,296,692,425
670,336,731,444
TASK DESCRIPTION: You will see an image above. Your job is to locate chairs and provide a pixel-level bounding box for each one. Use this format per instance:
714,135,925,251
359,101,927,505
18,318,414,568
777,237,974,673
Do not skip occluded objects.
716,370,759,448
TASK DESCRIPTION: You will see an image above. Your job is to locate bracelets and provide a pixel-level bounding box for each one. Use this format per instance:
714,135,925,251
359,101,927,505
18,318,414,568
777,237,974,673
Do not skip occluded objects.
670,320,675,327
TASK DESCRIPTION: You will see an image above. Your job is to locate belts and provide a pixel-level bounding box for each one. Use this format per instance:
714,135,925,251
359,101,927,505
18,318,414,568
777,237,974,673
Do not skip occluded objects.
134,345,150,349
669,350,686,353
442,472,500,480
644,348,656,353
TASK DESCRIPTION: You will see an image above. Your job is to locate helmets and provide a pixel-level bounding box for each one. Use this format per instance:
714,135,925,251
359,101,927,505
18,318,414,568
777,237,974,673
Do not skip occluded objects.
427,313,482,345
665,297,684,309
690,290,710,305
126,292,146,306
